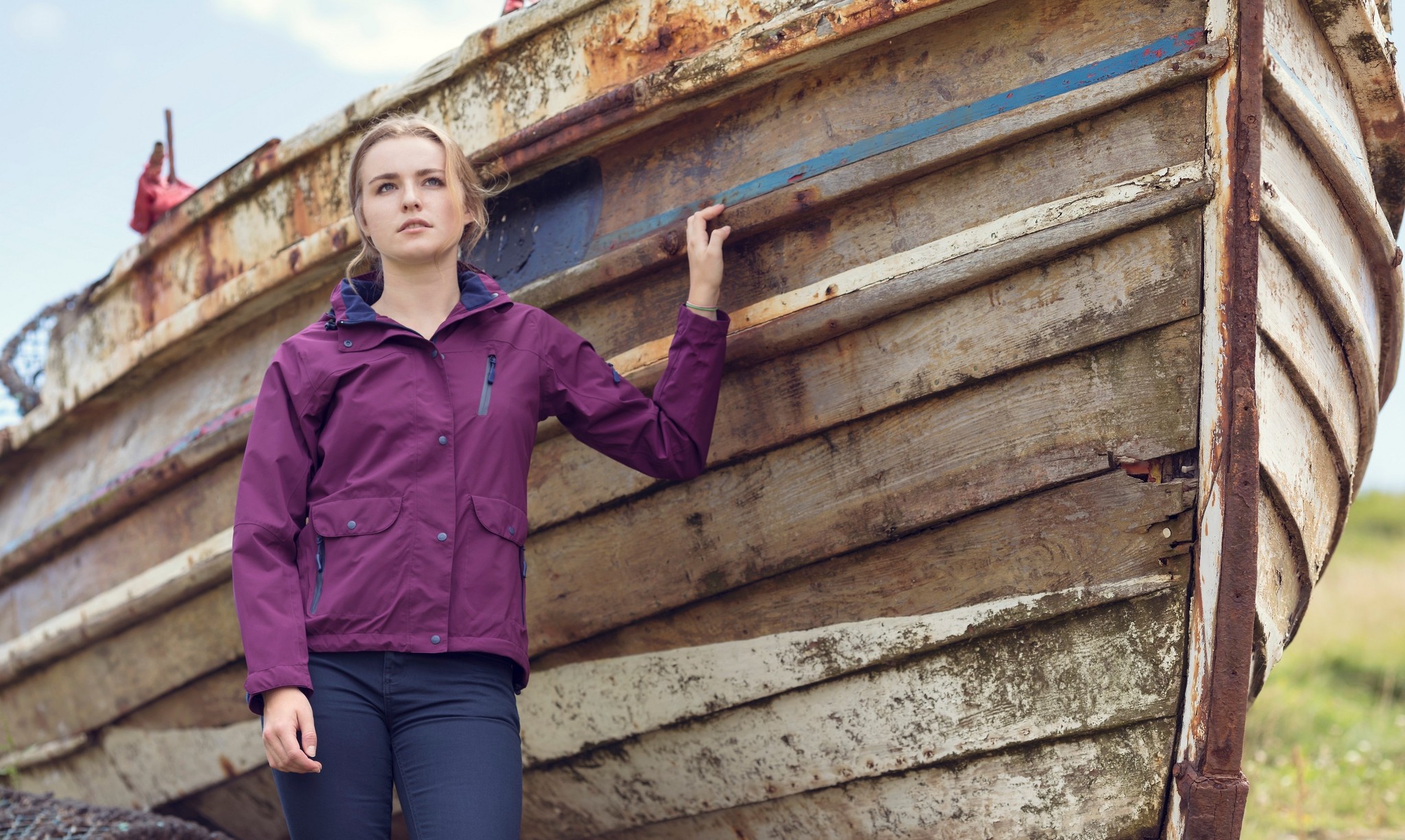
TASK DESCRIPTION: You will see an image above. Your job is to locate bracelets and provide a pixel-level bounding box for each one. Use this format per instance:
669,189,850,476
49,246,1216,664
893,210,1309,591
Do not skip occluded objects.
686,301,719,311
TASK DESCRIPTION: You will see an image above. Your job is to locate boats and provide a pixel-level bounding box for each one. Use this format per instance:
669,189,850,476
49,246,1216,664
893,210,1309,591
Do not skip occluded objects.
0,0,1405,840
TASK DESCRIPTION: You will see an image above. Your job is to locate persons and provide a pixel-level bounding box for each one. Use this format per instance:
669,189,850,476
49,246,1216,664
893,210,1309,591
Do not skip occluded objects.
230,115,732,840
